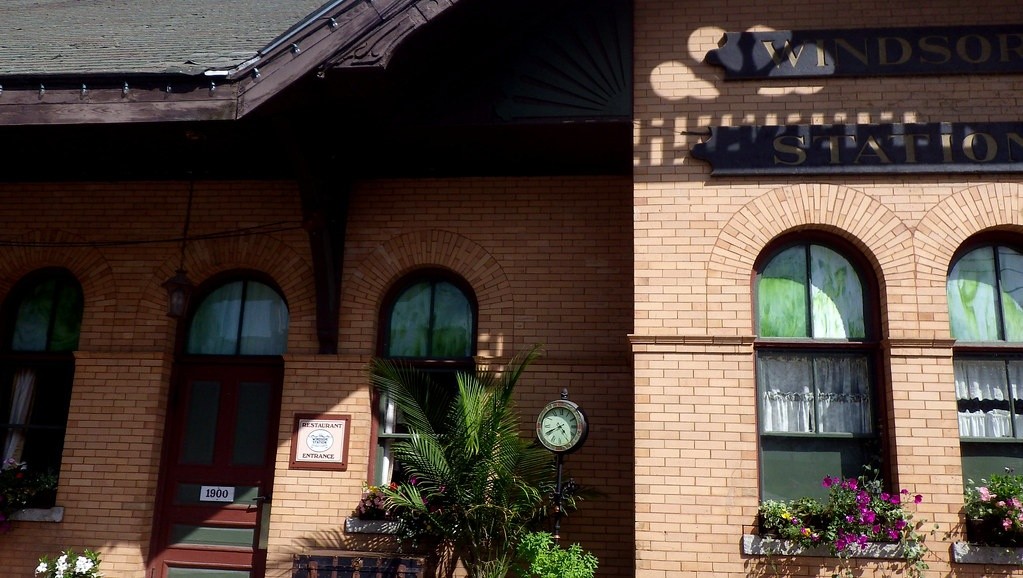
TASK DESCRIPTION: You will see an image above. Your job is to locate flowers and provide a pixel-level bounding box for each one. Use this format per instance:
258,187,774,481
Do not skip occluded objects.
358,478,434,515
0,457,58,535
966,467,1023,539
758,464,947,578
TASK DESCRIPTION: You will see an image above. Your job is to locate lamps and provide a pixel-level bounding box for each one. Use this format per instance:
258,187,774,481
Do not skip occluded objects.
160,268,196,320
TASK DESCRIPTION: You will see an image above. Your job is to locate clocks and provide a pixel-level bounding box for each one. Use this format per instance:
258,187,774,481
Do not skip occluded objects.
536,388,590,454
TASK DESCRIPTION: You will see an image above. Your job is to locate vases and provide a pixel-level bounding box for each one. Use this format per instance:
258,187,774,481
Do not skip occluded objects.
761,516,782,539
803,515,828,535
969,518,991,545
31,491,52,509
